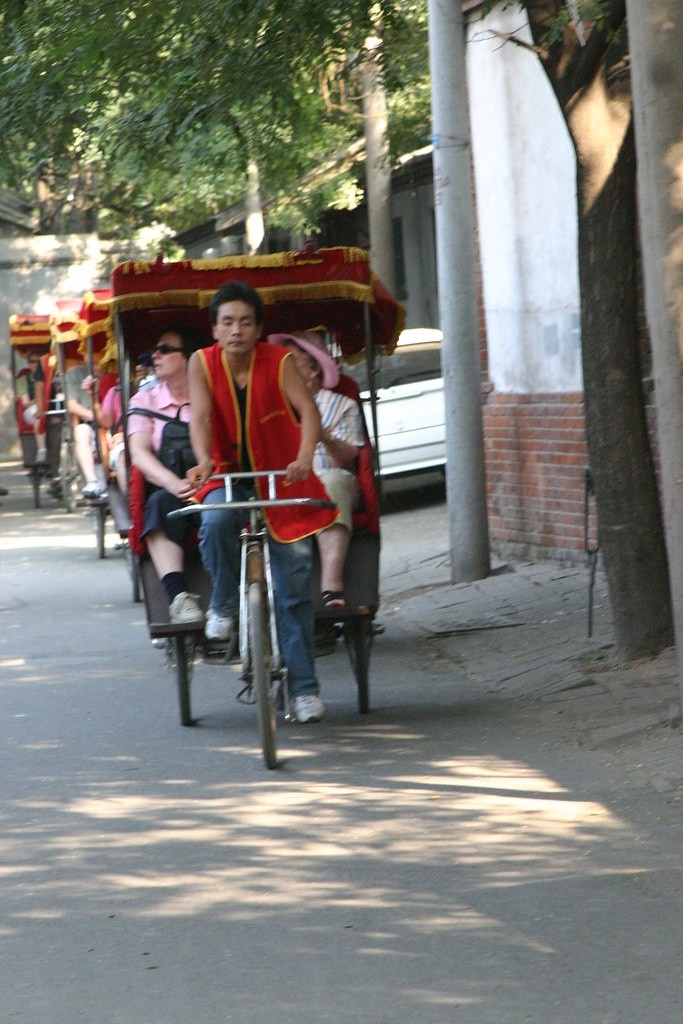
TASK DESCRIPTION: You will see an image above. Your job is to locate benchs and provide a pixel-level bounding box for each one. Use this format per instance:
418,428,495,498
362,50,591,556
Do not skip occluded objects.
130,373,383,550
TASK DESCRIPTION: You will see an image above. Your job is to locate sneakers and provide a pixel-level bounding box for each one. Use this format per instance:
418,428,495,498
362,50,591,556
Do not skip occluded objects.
169,591,203,624
292,694,328,723
204,609,233,641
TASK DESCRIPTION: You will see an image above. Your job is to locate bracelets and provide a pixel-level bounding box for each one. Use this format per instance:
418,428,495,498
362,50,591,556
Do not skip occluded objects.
326,433,337,450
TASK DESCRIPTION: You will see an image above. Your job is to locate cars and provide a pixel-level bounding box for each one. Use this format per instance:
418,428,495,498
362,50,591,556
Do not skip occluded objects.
315,329,445,505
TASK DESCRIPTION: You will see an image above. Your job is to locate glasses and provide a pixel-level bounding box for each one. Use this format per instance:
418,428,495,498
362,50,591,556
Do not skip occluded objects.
152,344,187,354
28,359,40,363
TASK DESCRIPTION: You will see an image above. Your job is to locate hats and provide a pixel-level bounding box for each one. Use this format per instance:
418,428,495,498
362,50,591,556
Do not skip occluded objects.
267,330,341,389
100,344,118,370
306,325,328,333
77,341,101,355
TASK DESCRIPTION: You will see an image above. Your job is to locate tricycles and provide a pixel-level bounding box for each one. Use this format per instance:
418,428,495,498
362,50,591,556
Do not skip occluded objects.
108,245,408,762
8,286,124,560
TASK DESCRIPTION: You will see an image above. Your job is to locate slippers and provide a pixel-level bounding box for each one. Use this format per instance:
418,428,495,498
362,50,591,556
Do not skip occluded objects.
322,589,346,607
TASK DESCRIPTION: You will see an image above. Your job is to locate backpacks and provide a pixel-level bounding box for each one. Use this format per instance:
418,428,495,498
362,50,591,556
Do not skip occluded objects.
124,407,199,479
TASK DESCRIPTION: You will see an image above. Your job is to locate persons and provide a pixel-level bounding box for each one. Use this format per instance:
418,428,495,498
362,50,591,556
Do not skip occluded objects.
63,363,108,498
267,327,364,609
126,327,204,625
186,282,340,724
16,350,49,464
79,370,131,518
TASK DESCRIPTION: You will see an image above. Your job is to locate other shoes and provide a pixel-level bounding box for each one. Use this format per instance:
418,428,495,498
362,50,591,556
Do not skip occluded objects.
81,481,102,498
34,450,46,464
151,638,168,648
